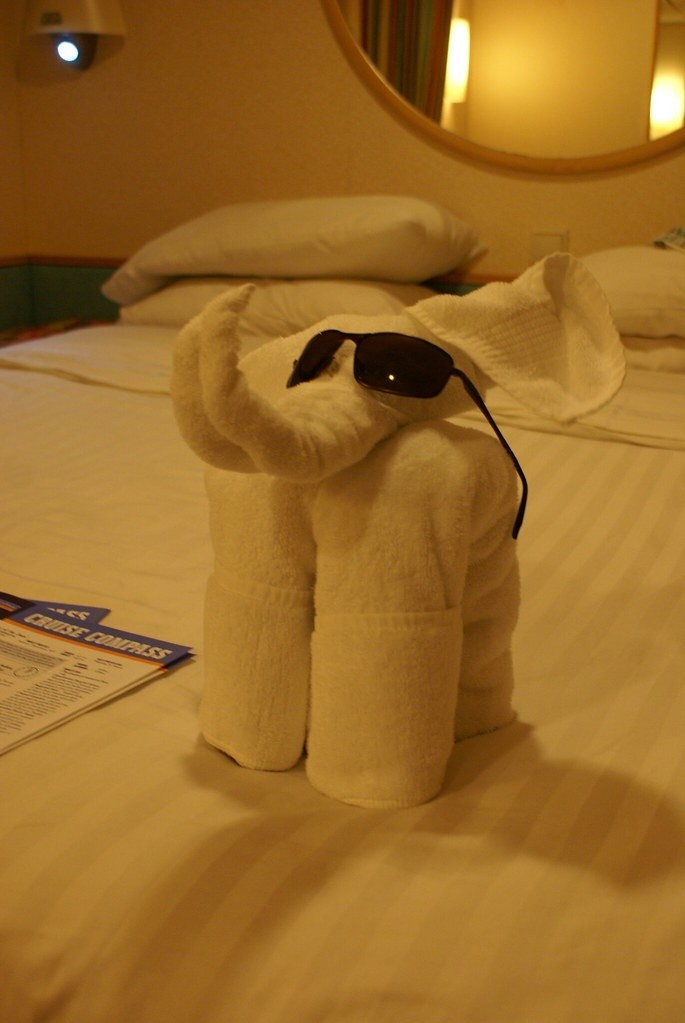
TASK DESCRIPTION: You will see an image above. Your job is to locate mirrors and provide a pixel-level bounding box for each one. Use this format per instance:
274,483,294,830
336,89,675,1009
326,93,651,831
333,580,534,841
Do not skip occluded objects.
322,0,685,176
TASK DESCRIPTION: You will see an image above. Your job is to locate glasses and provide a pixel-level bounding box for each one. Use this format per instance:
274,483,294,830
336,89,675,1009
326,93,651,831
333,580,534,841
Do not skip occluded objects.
286,329,528,539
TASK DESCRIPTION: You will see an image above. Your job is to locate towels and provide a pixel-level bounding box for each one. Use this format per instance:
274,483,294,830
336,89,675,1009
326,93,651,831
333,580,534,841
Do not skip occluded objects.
167,250,628,812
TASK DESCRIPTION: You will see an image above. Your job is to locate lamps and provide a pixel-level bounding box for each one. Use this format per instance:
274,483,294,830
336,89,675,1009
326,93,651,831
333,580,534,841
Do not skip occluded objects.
27,0,127,73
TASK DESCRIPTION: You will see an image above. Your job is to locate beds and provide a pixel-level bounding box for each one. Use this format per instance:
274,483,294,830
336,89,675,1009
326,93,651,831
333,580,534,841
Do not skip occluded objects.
0,321,685,1023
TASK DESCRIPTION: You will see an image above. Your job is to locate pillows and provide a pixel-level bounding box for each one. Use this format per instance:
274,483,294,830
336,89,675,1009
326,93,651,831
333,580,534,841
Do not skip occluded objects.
101,194,480,307
620,336,685,376
582,246,685,339
119,275,441,336
